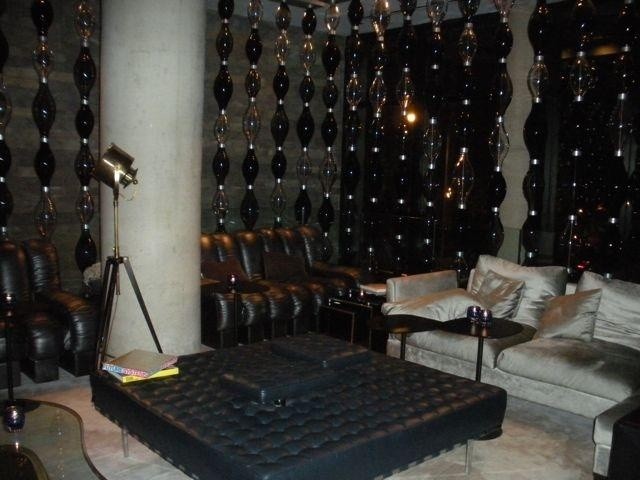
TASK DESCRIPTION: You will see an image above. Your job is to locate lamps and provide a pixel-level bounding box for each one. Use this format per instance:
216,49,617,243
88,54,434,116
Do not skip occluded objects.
88,142,164,200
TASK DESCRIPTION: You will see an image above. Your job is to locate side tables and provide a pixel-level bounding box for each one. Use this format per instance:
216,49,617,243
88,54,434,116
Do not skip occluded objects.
369,314,523,441
0,399,107,480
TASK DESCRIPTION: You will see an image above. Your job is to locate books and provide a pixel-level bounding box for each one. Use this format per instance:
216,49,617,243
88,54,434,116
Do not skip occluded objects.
359,282,386,293
360,287,386,297
102,349,179,379
105,363,179,384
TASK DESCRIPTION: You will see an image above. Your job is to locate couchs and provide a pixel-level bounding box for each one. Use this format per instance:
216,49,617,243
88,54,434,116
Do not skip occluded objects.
200,224,374,349
0,238,99,390
386,268,640,479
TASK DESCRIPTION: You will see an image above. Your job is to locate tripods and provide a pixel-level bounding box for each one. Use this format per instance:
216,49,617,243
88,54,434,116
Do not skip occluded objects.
92,199,163,403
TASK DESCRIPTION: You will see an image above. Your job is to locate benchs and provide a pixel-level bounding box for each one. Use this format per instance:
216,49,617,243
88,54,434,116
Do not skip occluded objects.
90,331,507,480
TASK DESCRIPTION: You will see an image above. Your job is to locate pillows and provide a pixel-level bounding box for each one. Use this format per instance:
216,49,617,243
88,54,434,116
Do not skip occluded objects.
380,253,640,353
